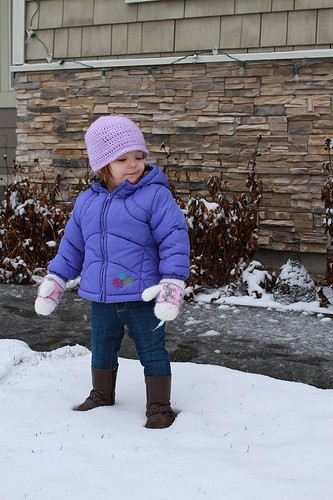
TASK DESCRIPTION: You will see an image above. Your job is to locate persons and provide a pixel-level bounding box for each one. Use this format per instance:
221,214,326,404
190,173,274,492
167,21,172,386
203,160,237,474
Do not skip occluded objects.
34,116,191,429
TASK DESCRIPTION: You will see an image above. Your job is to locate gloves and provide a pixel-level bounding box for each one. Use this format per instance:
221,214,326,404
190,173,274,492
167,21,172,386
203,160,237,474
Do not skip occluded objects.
142,279,186,321
35,274,65,315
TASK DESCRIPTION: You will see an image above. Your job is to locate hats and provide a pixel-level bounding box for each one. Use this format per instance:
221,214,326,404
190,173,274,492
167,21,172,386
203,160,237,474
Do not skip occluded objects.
84,116,149,173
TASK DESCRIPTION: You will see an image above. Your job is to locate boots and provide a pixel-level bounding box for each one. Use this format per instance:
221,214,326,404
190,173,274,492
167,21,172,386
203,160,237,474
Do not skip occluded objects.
144,374,176,429
74,364,119,410
